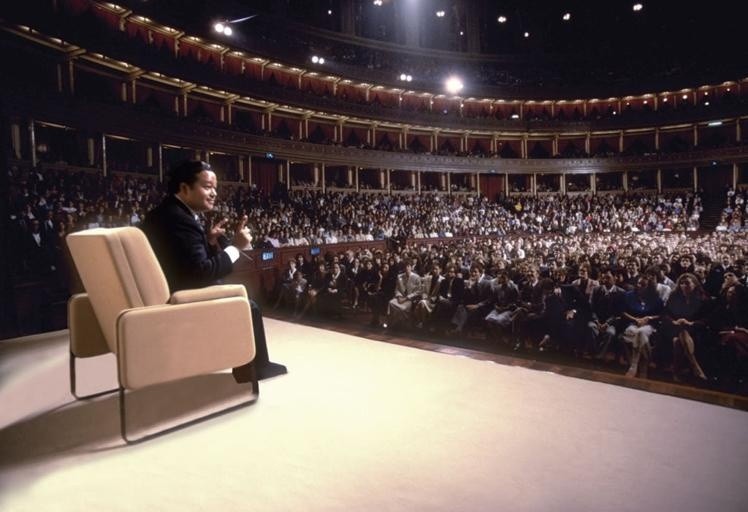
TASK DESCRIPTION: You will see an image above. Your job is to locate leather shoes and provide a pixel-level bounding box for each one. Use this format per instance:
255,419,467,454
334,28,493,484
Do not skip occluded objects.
233,361,287,384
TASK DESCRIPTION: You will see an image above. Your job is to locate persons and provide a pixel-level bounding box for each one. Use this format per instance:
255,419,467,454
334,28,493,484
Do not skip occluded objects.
0,153,746,396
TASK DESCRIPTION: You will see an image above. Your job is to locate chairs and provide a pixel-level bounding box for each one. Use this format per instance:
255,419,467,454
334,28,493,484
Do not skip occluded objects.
63,226,259,446
